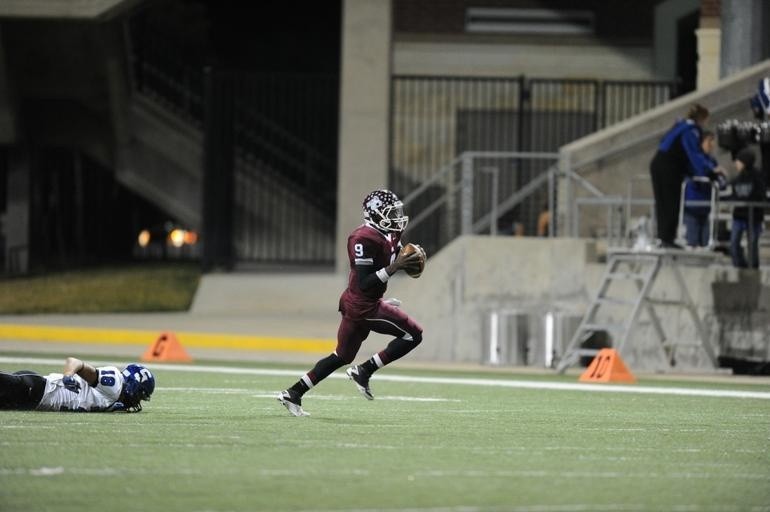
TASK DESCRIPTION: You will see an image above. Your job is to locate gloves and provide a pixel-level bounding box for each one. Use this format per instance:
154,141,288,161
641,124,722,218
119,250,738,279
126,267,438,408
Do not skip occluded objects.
62,375,82,394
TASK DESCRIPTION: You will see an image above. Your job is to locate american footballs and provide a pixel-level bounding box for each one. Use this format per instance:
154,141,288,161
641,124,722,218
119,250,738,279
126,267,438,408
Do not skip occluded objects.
403,242,425,278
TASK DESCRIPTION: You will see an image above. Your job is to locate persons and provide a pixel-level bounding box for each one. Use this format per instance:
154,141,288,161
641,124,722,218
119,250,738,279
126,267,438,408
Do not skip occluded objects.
650,76,770,270
276,190,426,418
0,356,156,417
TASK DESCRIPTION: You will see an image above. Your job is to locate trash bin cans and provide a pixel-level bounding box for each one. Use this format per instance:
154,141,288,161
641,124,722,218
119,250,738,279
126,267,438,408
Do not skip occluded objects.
544,312,585,369
479,309,530,368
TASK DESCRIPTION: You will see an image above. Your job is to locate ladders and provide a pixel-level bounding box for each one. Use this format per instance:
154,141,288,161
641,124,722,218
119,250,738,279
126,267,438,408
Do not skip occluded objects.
555,254,720,375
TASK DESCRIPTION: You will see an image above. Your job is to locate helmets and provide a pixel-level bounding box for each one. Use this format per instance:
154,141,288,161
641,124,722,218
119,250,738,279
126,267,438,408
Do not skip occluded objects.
363,189,409,233
118,364,154,413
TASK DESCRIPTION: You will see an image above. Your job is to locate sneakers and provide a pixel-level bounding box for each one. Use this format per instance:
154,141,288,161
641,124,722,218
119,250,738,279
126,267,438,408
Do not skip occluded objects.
278,390,311,417
346,365,374,401
